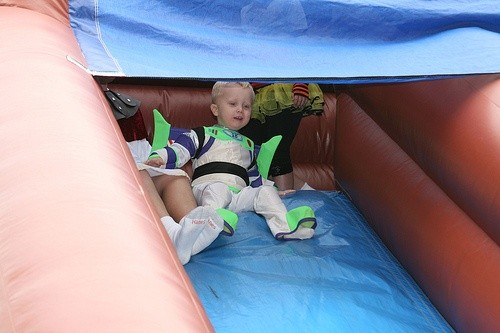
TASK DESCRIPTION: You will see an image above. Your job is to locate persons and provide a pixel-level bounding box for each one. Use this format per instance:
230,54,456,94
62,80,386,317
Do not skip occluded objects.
137,79,317,267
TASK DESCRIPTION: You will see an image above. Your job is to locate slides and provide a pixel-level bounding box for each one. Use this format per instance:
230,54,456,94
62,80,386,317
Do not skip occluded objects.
104,83,467,333
